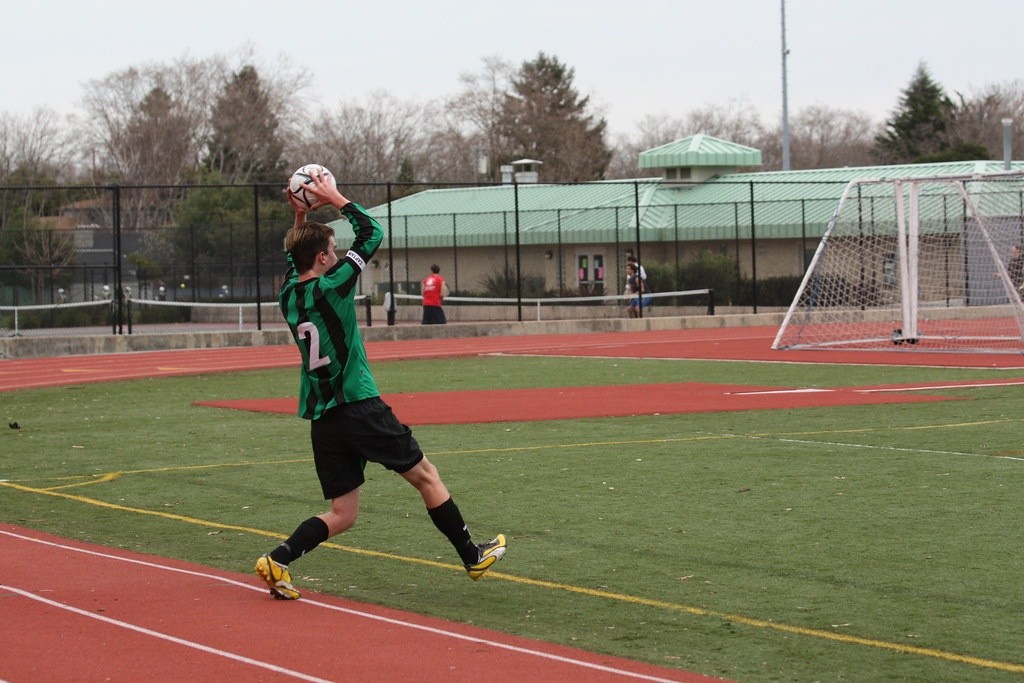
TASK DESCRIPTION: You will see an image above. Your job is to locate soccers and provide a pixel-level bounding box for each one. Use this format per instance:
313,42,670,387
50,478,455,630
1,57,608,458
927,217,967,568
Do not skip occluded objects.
289,163,337,212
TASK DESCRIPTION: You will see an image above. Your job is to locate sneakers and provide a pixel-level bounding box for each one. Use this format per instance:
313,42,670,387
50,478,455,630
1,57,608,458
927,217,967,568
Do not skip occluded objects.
255,552,301,599
462,533,507,580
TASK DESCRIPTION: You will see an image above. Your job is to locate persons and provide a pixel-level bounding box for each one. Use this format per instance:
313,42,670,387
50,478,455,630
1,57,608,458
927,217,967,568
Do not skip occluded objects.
421,265,449,324
993,243,1024,304
255,169,508,602
625,249,647,318
